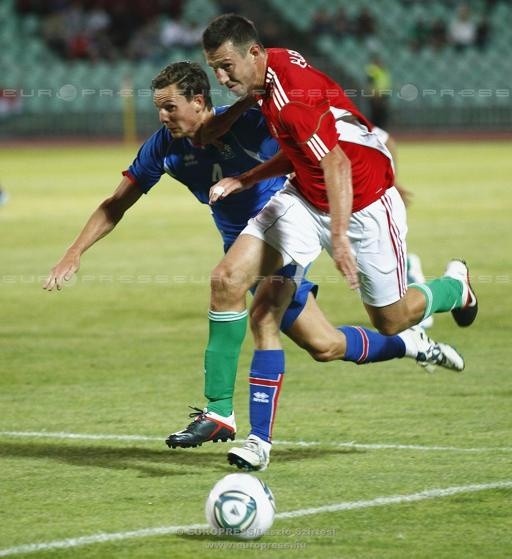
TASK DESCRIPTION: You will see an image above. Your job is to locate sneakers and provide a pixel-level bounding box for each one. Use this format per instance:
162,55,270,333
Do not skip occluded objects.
165,406,236,449
445,259,479,329
227,434,272,473
397,324,464,373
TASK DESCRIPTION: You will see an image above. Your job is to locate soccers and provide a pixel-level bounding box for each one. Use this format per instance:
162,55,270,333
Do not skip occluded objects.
206,473,275,540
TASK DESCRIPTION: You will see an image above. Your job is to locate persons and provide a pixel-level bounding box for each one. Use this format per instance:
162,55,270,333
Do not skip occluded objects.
448,3,482,48
39,0,211,67
41,13,479,472
